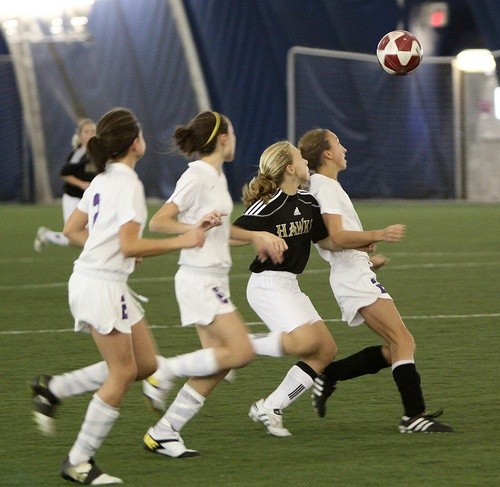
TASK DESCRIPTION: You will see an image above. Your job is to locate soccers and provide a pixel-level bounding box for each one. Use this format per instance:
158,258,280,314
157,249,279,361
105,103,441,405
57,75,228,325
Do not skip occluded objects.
376,30,424,76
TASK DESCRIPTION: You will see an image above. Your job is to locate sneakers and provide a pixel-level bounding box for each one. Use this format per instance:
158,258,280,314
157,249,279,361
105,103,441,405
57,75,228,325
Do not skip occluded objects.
224,368,234,383
311,372,336,418
27,373,61,437
60,455,123,485
398,408,454,433
247,398,293,436
143,426,200,458
142,355,167,414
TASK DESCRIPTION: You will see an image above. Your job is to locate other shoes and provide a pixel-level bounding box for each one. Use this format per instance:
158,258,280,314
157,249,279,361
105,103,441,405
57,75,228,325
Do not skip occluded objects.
34,226,49,253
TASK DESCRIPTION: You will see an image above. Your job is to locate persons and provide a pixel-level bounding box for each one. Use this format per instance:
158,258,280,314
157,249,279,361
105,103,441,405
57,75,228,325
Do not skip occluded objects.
228,140,376,440
298,128,453,433
34,118,98,253
27,107,205,487
141,111,283,457
